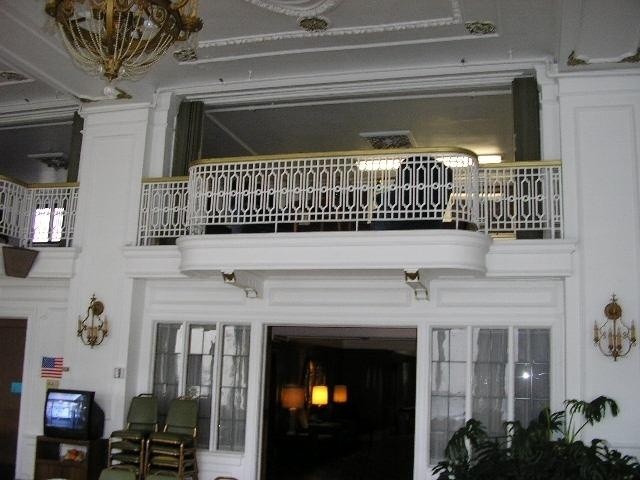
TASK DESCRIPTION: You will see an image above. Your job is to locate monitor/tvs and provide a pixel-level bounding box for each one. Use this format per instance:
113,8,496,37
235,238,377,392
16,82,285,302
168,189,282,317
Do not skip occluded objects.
43,388,105,439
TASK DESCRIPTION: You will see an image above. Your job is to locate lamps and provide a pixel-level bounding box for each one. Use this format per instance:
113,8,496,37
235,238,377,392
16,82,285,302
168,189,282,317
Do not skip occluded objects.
280,387,306,435
592,287,637,361
76,293,108,349
42,0,206,98
332,383,347,423
312,384,328,424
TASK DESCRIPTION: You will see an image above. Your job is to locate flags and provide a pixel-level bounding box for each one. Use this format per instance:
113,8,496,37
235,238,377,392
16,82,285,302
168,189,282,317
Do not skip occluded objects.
40,356,64,379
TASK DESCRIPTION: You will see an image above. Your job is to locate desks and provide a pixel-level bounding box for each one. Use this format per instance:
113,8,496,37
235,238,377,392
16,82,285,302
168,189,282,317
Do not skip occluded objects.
35,436,100,480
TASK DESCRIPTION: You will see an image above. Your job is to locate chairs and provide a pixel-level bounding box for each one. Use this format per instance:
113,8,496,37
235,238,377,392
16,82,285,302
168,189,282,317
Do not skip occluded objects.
98,393,202,480
373,156,454,219
